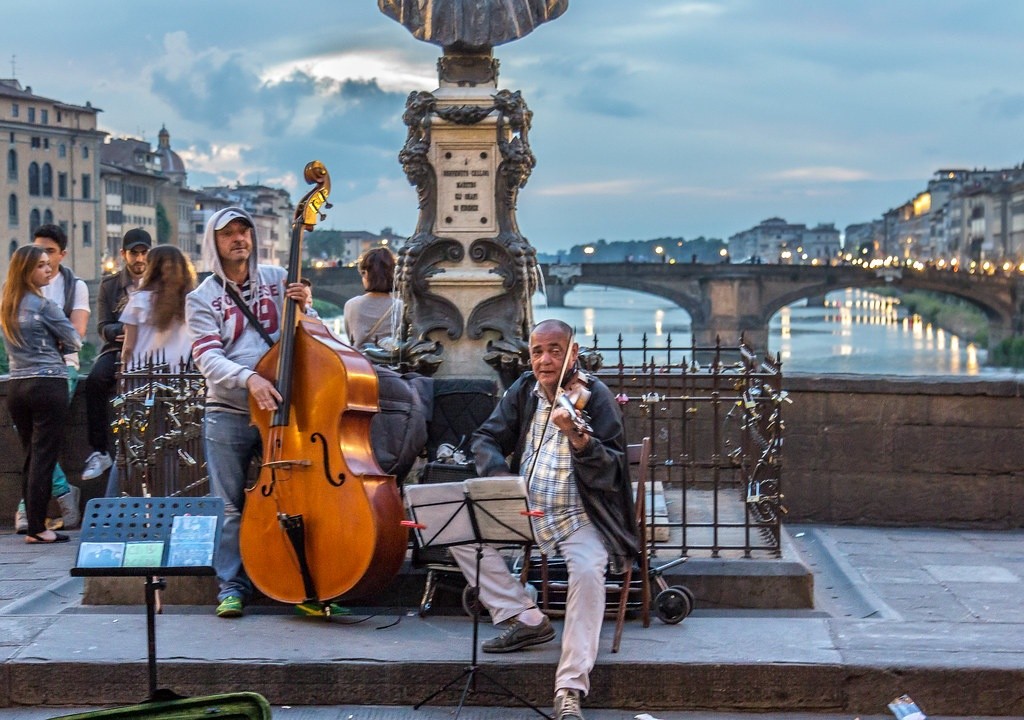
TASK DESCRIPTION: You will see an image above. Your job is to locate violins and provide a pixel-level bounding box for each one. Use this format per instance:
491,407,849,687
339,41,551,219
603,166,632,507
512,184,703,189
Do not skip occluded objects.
549,369,593,444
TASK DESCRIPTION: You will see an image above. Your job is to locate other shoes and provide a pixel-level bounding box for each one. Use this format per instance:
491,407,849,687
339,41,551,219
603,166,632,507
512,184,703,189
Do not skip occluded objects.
26,528,71,543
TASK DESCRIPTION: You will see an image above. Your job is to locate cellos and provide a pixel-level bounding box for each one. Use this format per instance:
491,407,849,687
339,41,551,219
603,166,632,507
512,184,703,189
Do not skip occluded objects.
231,160,413,618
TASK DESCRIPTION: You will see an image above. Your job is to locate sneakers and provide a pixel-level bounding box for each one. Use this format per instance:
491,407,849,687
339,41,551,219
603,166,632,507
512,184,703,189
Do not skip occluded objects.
482,614,556,653
216,596,242,616
57,484,79,528
80,451,112,480
297,601,351,618
16,511,28,533
553,688,584,720
45,517,63,530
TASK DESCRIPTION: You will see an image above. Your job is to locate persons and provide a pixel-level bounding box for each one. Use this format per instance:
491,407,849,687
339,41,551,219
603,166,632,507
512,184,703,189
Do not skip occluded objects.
82,229,154,480
451,320,641,720
103,245,198,497
183,207,351,621
298,278,314,308
12,224,91,534
0,245,83,543
343,247,405,348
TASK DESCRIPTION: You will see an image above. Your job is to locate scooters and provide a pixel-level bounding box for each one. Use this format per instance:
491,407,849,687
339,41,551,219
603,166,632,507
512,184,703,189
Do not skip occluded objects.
462,546,696,626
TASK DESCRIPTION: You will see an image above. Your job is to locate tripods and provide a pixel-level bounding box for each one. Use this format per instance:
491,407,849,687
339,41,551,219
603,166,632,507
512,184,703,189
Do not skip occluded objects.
410,489,557,720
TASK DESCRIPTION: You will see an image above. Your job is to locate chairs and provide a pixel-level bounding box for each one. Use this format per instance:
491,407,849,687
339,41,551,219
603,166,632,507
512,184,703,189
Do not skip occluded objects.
521,437,650,653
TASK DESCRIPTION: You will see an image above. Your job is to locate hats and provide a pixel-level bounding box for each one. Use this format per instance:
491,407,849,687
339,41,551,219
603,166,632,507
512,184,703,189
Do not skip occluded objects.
215,210,253,230
122,228,151,250
